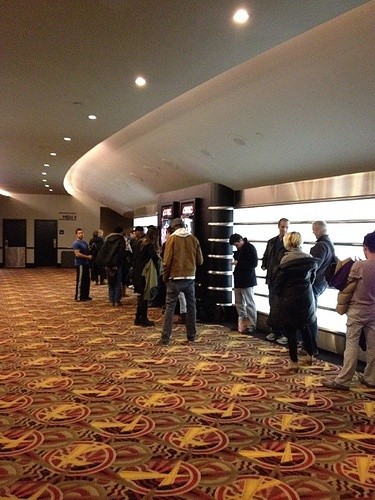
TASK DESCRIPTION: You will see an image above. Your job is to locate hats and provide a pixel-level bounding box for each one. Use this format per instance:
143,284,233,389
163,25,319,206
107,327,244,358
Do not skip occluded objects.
172,219,185,226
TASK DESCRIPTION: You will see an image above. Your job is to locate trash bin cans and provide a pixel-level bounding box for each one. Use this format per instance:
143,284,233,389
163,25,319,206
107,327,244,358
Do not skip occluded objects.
61,251,75,268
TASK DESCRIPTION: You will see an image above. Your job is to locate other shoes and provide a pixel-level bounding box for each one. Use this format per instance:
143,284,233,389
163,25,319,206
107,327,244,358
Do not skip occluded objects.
266,333,276,341
283,361,299,373
123,293,130,297
134,321,154,327
360,376,375,388
307,356,317,365
96,282,98,285
323,380,349,391
100,281,107,285
188,335,195,343
277,337,289,345
75,297,92,301
157,339,169,347
109,302,120,307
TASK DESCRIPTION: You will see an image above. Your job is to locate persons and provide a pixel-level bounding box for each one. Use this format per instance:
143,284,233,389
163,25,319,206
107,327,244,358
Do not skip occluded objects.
88,225,199,326
272,231,323,371
131,225,160,327
324,230,375,392
155,218,204,347
229,233,258,335
261,218,289,345
72,227,93,302
297,221,336,357
